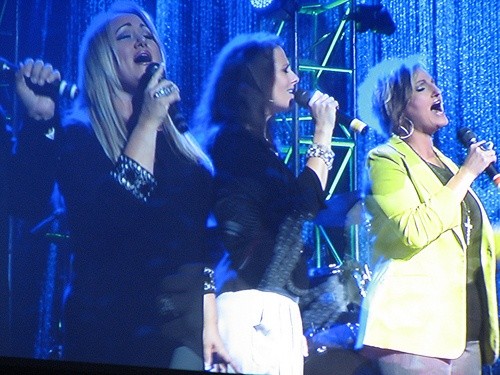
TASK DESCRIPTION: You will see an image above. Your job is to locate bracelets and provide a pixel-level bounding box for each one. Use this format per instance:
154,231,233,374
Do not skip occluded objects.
306,143,335,170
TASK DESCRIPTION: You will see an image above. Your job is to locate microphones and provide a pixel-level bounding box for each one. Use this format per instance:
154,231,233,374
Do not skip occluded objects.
0,56,81,99
293,87,370,135
457,127,500,185
146,61,189,133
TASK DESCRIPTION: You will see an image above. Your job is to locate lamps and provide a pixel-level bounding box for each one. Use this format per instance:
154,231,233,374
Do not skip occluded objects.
250,0,299,23
355,1,397,36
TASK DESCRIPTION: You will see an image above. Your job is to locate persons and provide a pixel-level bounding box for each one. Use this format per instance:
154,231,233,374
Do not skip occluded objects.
190,31,339,375
356,56,500,375
51,0,242,369
0,58,62,219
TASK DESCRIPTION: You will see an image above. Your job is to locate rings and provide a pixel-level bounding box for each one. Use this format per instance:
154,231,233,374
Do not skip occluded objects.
480,143,491,150
155,84,173,99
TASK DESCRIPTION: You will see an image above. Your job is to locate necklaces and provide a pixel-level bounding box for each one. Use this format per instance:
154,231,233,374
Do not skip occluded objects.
409,144,473,245
